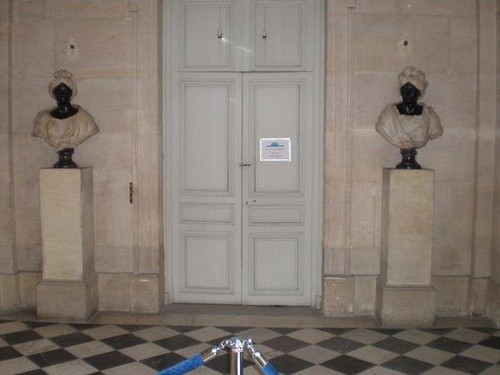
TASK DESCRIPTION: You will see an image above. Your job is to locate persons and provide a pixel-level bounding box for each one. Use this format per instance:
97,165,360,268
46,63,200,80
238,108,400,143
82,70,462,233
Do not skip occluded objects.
30,68,100,168
377,65,444,171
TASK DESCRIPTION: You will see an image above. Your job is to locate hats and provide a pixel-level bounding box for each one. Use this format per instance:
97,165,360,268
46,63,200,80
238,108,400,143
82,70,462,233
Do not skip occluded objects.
49,68,77,99
398,64,426,96
